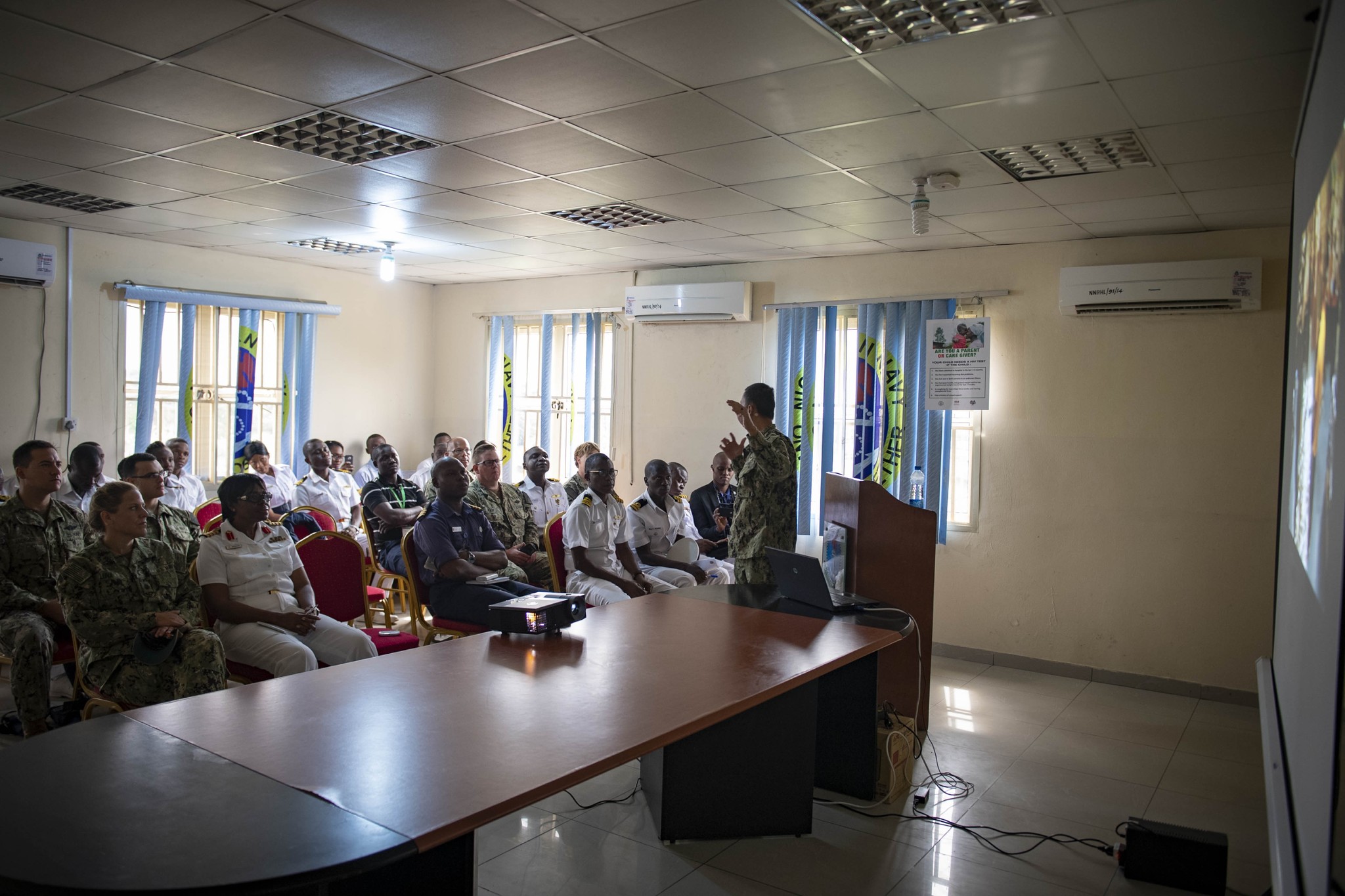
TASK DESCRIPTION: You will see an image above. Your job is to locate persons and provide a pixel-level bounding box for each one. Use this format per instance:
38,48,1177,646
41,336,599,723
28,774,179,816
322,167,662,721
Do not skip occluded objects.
291,439,372,558
324,432,497,503
0,438,207,516
965,324,984,348
563,441,619,506
0,439,98,740
690,451,738,560
561,453,678,607
668,462,736,584
119,452,201,564
195,472,378,679
625,459,730,587
720,383,798,585
57,481,228,710
359,444,431,580
245,440,297,521
464,445,555,594
513,446,569,552
412,456,553,629
952,323,967,349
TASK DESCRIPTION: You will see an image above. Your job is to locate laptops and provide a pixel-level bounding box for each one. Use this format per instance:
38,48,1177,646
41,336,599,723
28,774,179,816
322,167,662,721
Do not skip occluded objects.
765,545,881,613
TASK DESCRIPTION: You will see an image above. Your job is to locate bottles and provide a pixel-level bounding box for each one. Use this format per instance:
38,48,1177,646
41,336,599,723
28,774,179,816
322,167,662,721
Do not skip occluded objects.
910,466,927,508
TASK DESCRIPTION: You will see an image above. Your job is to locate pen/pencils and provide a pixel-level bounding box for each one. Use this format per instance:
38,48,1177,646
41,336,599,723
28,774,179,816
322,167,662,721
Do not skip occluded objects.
706,574,719,578
305,604,317,616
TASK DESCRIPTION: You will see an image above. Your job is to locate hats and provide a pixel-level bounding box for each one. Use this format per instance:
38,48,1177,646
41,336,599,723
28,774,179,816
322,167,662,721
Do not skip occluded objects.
666,538,699,564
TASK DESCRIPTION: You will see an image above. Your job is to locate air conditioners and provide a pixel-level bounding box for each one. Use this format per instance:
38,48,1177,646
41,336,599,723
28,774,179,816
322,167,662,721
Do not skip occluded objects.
624,280,754,325
0,236,58,289
1057,256,1265,317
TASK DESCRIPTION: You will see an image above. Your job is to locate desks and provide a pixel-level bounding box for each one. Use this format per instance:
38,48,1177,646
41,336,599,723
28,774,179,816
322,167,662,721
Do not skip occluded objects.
0,579,918,896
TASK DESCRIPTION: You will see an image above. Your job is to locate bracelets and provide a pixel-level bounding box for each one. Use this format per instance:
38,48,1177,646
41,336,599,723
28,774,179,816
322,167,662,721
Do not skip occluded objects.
306,604,321,615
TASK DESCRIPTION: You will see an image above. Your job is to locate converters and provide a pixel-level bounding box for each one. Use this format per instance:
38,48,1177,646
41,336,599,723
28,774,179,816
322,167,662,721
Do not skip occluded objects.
915,785,930,805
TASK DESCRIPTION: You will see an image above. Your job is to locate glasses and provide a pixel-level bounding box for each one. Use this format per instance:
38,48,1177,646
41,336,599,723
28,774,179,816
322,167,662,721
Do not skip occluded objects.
477,458,503,466
132,470,168,480
230,493,273,503
448,447,472,454
588,470,618,477
333,455,346,461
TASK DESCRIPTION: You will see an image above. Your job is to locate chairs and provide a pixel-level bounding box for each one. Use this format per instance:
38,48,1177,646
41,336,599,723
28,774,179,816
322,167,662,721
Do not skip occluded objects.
399,525,494,647
70,629,146,723
277,504,392,629
294,530,420,657
544,510,568,593
201,512,224,536
359,505,434,637
188,556,332,686
0,647,79,728
191,496,223,534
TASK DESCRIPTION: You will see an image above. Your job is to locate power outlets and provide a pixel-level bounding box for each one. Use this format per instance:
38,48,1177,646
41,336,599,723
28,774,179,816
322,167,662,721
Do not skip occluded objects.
61,416,78,432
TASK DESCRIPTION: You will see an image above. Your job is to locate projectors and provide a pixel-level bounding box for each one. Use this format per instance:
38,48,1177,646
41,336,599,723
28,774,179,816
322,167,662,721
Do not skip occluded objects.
488,591,587,635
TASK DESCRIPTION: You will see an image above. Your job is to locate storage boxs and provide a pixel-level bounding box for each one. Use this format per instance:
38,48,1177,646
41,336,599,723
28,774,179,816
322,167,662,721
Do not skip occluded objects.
875,712,915,805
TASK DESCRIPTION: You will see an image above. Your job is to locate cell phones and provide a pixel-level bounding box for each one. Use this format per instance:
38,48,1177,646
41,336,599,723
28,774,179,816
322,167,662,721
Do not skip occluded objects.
378,630,400,636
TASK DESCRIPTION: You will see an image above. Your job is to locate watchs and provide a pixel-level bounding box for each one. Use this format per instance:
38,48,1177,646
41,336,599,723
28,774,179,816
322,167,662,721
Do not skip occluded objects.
350,525,360,535
632,570,646,580
465,550,476,564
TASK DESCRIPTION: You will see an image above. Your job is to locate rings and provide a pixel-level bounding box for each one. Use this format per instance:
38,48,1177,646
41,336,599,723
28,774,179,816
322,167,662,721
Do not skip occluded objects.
346,530,349,533
740,406,744,410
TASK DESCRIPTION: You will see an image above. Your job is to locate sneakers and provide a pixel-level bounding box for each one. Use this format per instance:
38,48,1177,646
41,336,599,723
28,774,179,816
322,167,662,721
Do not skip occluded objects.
356,608,399,624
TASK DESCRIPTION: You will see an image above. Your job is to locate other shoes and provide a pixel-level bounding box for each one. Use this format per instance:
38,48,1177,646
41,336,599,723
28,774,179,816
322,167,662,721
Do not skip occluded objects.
23,719,48,739
